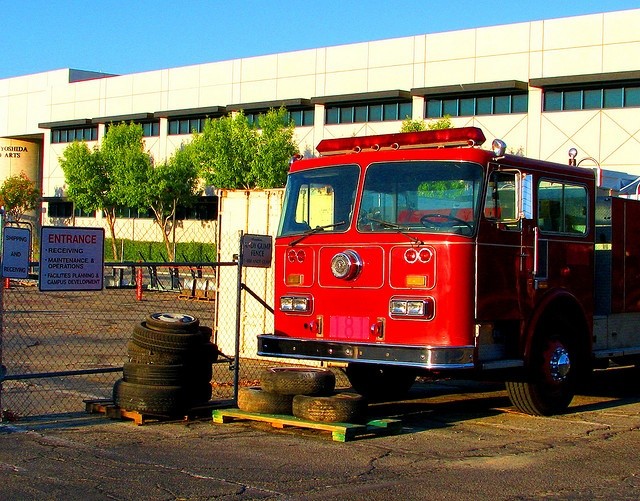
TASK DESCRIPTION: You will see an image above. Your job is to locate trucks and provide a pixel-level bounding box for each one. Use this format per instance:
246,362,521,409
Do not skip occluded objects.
258,128,640,416
